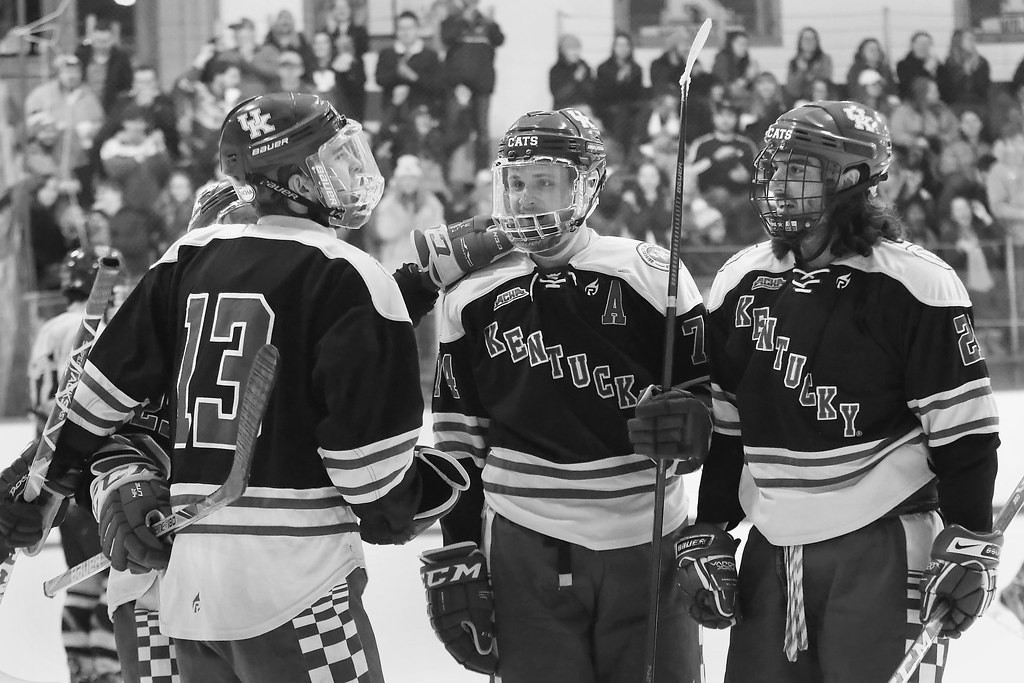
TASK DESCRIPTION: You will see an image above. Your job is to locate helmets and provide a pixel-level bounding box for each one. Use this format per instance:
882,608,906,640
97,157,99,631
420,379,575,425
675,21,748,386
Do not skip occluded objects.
60,245,125,298
186,177,259,233
218,93,385,230
490,108,607,254
748,99,892,236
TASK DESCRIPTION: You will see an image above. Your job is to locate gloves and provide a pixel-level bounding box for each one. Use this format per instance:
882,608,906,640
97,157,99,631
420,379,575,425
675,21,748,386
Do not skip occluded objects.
89,453,175,574
672,524,741,627
0,447,44,555
918,524,1004,639
420,539,500,676
628,383,714,475
410,212,517,292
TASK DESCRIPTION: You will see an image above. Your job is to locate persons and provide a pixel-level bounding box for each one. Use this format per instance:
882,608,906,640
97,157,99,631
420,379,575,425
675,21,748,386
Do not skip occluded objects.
546,27,1024,320
0,94,470,683
0,0,507,281
709,97,1003,683
420,110,707,683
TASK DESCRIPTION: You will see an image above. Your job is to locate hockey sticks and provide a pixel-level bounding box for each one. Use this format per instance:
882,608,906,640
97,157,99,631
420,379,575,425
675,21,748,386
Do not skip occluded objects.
886,474,1024,683
39,341,282,603
0,253,123,604
641,16,714,683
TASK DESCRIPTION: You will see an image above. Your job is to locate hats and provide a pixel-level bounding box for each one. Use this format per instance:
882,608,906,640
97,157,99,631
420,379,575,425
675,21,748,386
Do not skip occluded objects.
393,154,421,177
859,70,886,85
278,52,302,66
